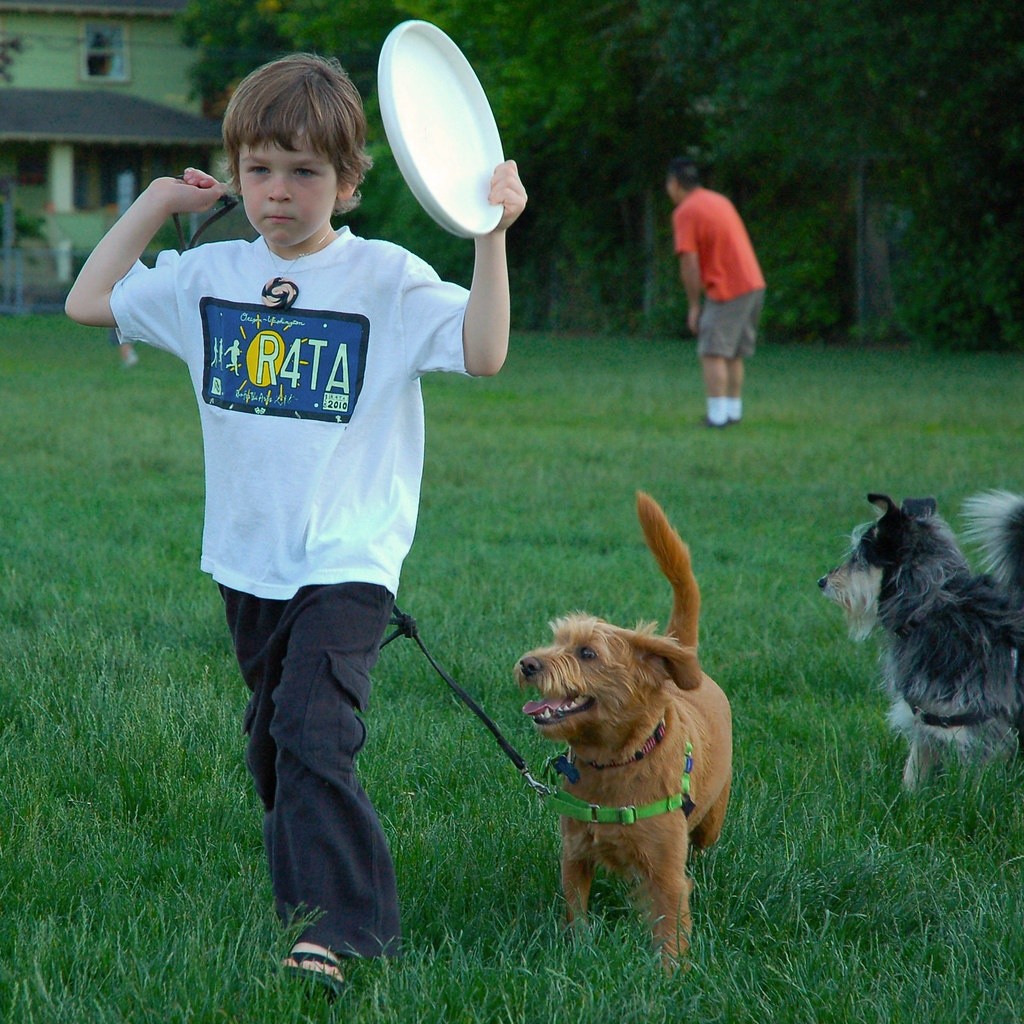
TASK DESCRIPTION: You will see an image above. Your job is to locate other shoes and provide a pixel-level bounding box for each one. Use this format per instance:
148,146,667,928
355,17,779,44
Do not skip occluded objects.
698,414,741,429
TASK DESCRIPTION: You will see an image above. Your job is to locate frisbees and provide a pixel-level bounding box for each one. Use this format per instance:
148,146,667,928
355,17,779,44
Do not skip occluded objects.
377,19,506,237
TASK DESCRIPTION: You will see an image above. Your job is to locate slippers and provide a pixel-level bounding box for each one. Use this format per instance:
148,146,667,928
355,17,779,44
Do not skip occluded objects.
274,951,344,1005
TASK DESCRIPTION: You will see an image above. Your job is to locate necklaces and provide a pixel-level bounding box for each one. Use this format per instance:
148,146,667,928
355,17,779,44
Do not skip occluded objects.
262,227,332,310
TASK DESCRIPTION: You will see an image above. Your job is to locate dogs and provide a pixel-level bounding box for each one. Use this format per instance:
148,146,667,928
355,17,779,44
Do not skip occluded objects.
818,491,1024,795
511,490,733,976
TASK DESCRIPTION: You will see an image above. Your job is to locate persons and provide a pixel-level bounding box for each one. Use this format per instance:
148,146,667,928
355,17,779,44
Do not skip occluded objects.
665,159,767,430
64,54,528,1003
110,324,139,367
